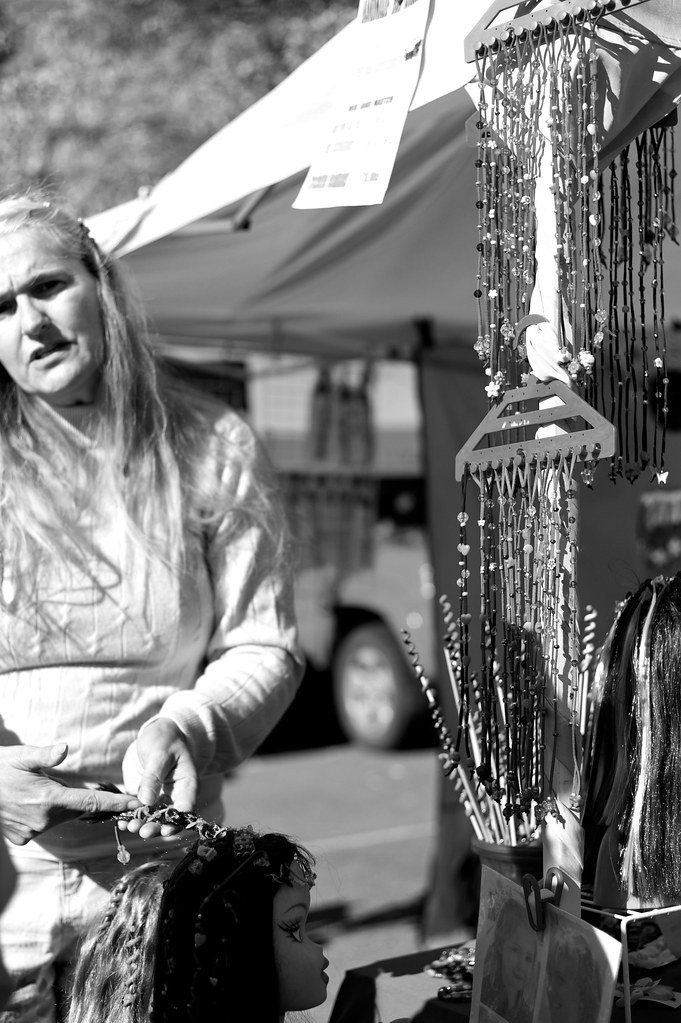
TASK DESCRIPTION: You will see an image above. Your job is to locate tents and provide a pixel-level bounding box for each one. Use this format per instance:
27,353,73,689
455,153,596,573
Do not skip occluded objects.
77,0,681,371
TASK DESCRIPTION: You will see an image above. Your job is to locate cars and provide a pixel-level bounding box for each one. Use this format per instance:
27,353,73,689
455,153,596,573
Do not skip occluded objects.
270,481,440,754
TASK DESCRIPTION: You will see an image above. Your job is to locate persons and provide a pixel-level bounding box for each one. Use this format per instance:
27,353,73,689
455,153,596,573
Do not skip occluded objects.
65,782,329,1023
0,194,309,1023
